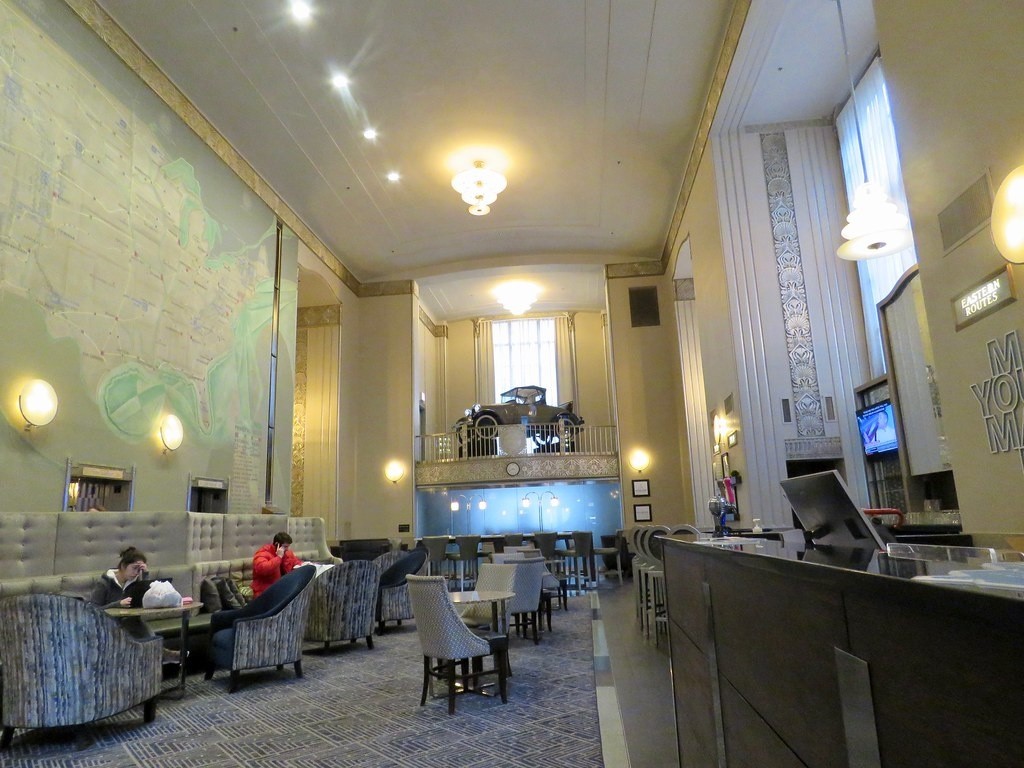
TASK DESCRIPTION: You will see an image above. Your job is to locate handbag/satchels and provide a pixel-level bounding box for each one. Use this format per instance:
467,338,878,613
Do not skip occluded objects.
142,579,184,607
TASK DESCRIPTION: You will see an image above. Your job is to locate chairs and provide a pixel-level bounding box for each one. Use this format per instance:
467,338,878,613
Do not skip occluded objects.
0,595,164,751
489,546,560,646
204,565,317,695
423,528,625,596
303,559,381,657
405,573,509,716
371,550,430,636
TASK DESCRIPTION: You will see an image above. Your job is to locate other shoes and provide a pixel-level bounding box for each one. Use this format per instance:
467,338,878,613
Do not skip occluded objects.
161,649,189,664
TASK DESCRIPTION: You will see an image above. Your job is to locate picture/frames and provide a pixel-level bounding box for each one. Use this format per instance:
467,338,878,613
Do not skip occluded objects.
631,479,651,498
633,504,653,523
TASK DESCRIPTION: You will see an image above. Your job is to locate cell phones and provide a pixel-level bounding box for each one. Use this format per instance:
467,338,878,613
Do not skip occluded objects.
275,542,281,548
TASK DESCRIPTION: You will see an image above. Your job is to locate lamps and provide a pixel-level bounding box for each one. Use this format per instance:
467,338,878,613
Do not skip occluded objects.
450,493,487,535
628,449,650,474
988,163,1024,261
836,181,914,261
451,159,508,217
713,414,726,447
17,379,59,432
492,287,543,317
159,414,183,456
384,461,404,486
521,490,559,532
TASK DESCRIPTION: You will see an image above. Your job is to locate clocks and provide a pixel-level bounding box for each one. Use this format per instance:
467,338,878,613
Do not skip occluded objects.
505,461,520,476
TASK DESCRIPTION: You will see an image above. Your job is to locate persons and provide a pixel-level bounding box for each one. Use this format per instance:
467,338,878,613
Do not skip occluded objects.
863,411,896,445
91,546,189,664
252,532,302,599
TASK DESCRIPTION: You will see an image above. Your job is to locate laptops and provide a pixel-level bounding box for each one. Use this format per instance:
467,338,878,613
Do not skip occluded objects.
117,577,173,608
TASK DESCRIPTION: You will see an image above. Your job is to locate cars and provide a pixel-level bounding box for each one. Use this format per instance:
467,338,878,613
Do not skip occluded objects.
453,384,585,447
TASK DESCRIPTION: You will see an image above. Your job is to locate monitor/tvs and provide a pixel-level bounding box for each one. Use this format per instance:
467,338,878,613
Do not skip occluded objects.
856,398,899,461
779,469,887,553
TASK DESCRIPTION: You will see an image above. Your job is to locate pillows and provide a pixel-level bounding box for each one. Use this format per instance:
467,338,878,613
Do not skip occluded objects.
200,576,248,613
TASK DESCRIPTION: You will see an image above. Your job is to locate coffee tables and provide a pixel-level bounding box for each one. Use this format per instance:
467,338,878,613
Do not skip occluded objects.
103,601,203,702
448,590,516,682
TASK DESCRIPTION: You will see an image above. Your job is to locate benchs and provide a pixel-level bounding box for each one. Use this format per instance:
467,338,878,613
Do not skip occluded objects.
0,510,344,643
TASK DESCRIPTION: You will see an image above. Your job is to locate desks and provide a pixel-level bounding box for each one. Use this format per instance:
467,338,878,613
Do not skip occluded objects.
413,531,597,592
